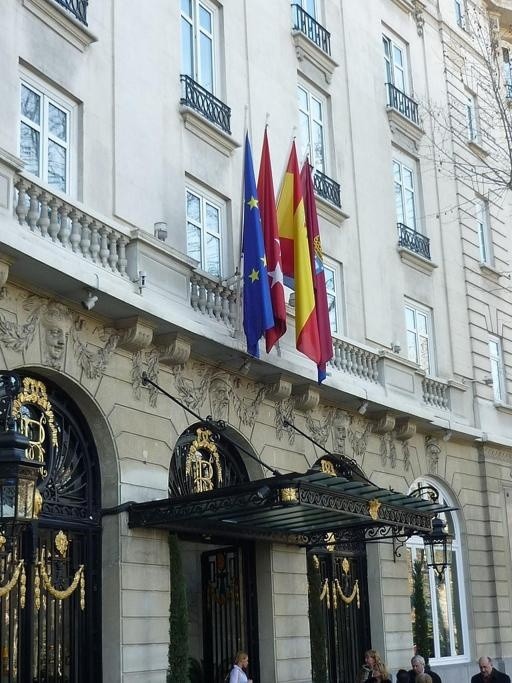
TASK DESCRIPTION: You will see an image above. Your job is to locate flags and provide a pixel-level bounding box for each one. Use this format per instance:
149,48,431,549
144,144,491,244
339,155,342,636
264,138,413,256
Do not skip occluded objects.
300,157,334,386
243,130,274,357
276,139,322,365
255,129,288,353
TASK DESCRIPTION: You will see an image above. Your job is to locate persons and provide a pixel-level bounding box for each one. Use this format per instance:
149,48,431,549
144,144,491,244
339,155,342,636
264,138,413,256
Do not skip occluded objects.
356,649,441,682
229,651,254,683
470,655,510,682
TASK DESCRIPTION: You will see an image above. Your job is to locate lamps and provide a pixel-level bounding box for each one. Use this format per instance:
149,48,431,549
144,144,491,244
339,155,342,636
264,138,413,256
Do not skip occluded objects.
0,415,47,553
461,374,494,386
239,357,252,375
358,399,368,414
153,221,168,242
379,340,401,354
82,291,99,310
390,486,456,581
442,430,452,441
